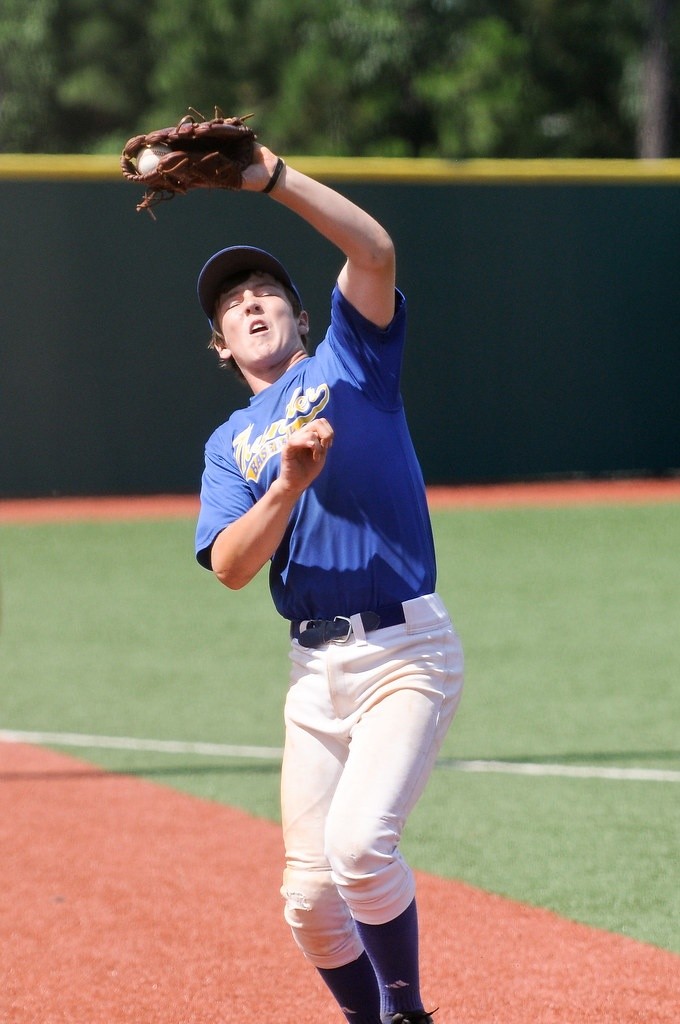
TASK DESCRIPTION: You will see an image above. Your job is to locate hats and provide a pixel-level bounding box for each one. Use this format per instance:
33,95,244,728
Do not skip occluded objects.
195,245,303,330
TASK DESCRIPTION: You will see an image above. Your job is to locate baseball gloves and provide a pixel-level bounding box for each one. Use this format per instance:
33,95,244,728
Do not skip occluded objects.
120,105,256,213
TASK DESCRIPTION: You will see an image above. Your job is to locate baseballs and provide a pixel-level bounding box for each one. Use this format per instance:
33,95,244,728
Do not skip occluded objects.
134,145,172,178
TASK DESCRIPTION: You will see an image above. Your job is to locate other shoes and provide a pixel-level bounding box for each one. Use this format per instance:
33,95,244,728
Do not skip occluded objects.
381,1006,440,1024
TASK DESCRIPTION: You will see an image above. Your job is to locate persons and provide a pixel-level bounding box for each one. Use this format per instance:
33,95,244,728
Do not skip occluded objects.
119,106,464,1023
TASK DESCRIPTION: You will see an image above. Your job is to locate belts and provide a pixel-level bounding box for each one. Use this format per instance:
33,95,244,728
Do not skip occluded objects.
290,605,406,643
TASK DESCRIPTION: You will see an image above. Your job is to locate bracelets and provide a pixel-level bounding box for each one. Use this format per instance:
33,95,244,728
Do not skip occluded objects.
259,158,285,194
